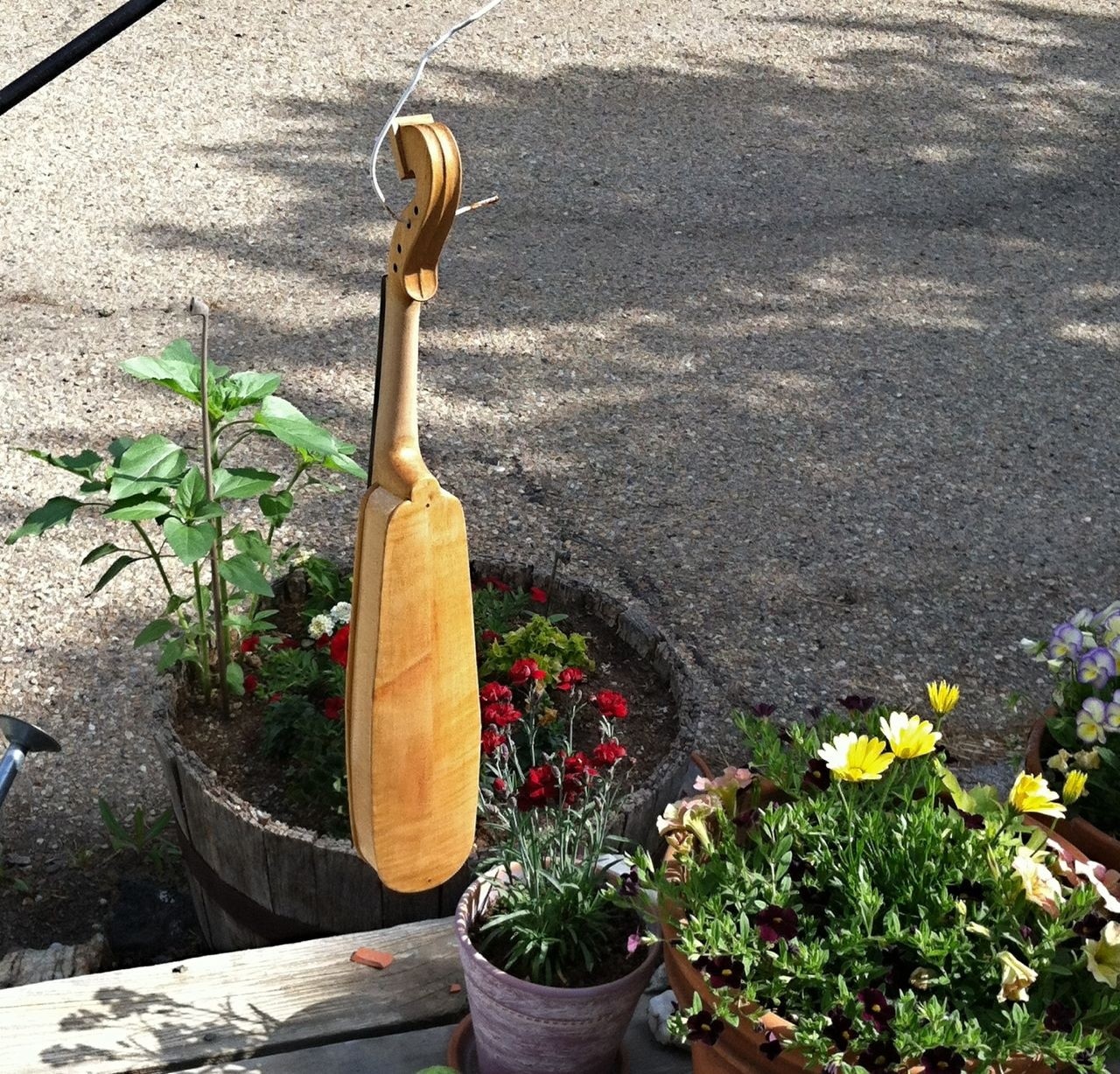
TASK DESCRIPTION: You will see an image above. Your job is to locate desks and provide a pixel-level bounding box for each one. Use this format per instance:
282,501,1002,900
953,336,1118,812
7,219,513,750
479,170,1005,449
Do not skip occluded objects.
0,917,692,1074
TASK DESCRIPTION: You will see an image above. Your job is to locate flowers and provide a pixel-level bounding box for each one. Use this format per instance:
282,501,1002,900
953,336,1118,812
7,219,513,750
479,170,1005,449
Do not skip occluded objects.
241,563,1120,1074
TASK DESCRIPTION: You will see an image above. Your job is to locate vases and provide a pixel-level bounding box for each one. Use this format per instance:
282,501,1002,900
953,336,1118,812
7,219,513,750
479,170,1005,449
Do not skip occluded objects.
153,553,699,964
455,856,662,1074
1025,705,1120,872
659,811,1120,1074
